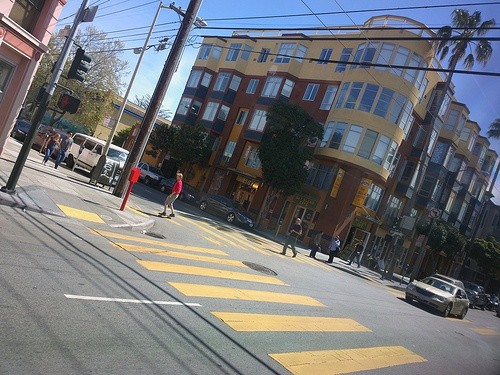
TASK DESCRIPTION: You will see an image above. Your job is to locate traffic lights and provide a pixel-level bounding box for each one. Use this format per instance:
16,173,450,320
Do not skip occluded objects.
66,47,93,83
59,93,81,114
392,216,399,230
384,234,392,242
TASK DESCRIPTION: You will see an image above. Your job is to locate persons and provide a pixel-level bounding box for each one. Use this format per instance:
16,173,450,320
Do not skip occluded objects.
309,231,324,258
279,218,302,257
158,173,183,217
41,133,61,165
37,129,54,156
347,240,364,269
327,235,341,263
54,131,74,170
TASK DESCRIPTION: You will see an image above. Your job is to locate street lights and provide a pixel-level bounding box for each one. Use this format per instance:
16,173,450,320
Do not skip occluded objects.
88,1,208,186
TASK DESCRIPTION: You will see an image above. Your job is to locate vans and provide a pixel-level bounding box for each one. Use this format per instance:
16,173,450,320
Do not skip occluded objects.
63,131,131,184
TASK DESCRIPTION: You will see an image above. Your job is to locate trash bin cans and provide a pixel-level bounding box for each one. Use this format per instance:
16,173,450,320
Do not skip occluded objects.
90,154,120,191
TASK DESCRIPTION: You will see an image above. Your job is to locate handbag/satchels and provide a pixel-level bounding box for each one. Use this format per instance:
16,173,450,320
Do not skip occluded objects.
336,247,340,251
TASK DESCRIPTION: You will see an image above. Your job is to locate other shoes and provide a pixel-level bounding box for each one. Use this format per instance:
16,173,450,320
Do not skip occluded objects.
159,212,166,216
346,263,350,265
326,261,328,262
170,213,175,217
293,253,297,257
328,262,331,263
280,253,285,255
312,256,316,259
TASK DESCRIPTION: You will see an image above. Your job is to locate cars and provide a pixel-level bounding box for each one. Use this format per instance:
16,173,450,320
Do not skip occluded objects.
462,279,487,311
11,117,69,159
199,192,257,230
135,161,162,188
433,273,465,290
158,178,199,202
486,293,500,316
405,276,470,320
